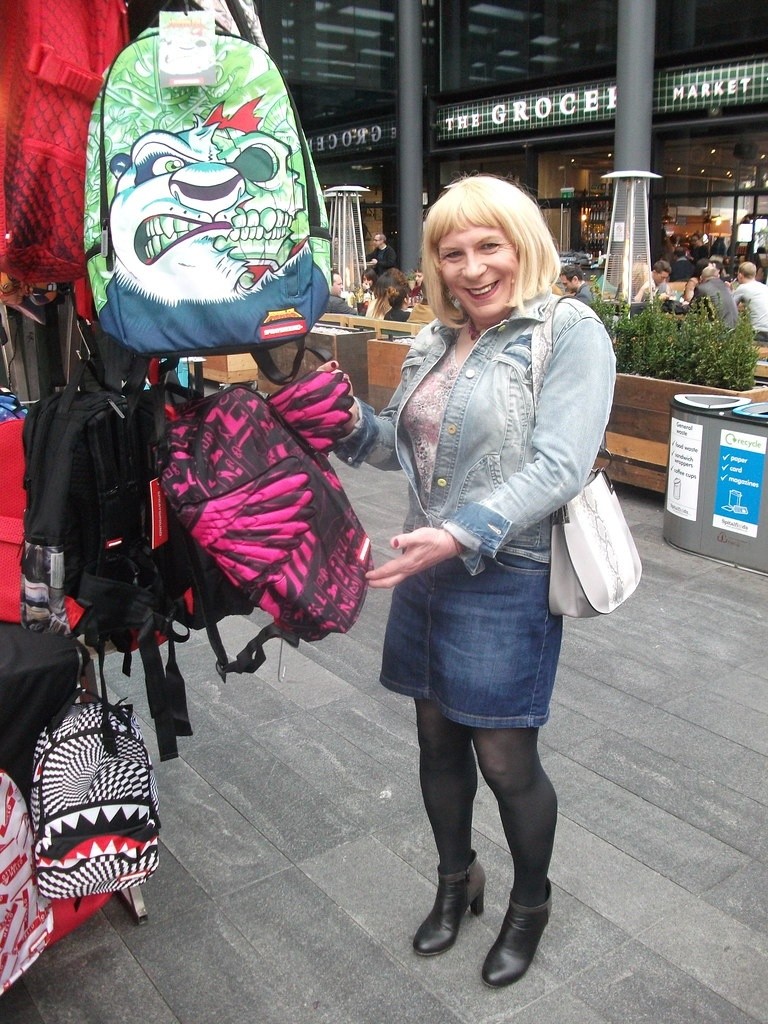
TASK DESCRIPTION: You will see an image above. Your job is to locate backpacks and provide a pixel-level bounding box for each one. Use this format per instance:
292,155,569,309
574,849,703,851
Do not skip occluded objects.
0,1,131,286
82,21,332,385
0,321,374,759
29,686,162,898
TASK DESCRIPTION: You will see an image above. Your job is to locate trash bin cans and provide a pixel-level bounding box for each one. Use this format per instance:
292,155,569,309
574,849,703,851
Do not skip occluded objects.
660,392,768,577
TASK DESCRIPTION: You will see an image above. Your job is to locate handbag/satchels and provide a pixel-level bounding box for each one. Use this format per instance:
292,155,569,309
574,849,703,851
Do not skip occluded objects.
532,296,645,621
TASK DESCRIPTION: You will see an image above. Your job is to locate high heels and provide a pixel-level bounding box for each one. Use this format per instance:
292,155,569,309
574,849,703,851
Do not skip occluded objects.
481,879,553,990
412,848,487,958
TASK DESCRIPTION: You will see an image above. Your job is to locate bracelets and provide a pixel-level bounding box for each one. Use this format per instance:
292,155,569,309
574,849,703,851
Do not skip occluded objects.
453,537,467,555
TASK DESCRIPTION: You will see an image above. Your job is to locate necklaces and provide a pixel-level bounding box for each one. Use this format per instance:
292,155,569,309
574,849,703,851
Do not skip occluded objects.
467,319,481,340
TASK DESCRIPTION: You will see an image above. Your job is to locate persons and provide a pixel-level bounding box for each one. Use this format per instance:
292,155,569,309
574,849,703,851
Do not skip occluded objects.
365,233,398,277
559,263,594,306
313,176,615,989
359,266,438,325
730,264,768,342
323,269,359,316
651,233,768,331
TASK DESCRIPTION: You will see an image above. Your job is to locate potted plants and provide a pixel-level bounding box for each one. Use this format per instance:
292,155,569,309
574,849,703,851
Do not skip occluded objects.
581,274,755,495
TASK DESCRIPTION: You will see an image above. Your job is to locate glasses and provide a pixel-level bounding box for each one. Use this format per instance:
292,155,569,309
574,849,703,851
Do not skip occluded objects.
691,239,699,242
